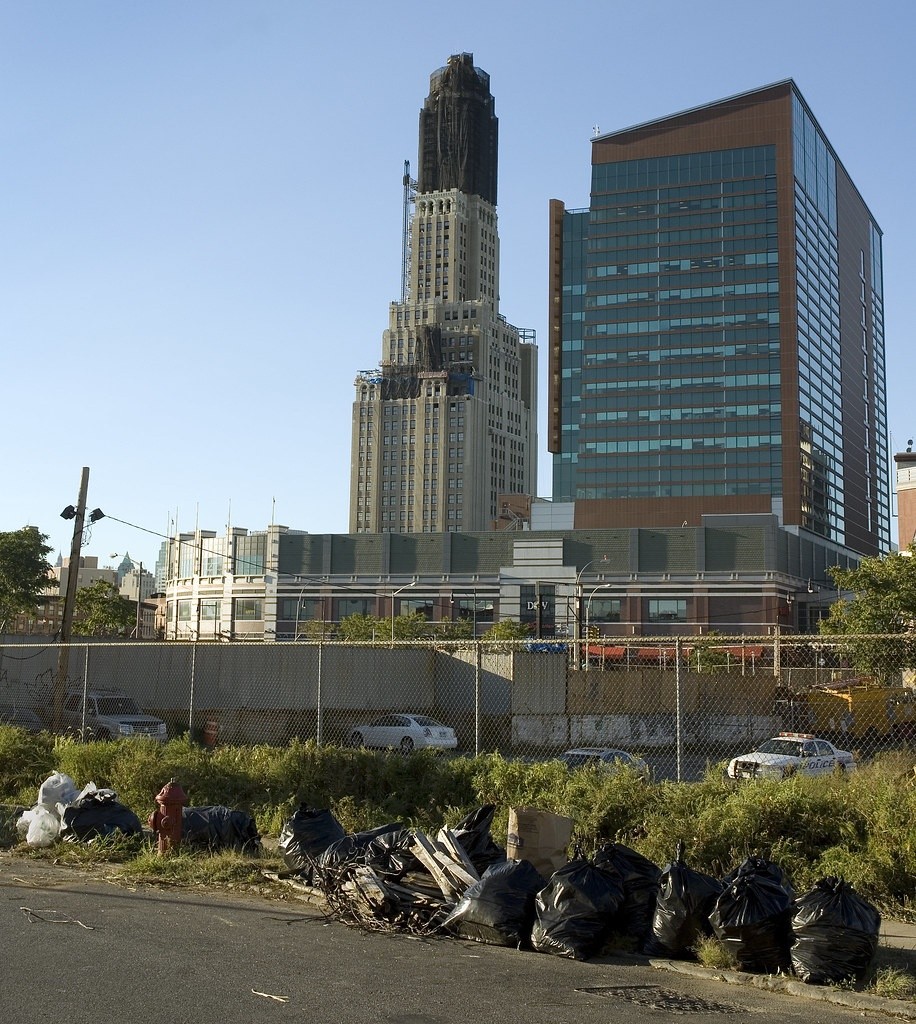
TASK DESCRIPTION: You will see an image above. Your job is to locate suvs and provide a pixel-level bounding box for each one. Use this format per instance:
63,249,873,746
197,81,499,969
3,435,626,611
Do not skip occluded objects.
44,689,170,743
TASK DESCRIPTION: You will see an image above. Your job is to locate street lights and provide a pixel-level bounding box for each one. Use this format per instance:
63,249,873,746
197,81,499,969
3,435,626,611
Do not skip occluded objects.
575,557,611,666
108,553,142,638
294,579,323,641
196,605,217,643
51,504,106,735
392,582,417,641
586,584,611,670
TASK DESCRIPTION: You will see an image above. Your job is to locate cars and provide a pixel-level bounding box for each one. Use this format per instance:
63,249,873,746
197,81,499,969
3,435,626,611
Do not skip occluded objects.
727,731,858,781
545,748,651,785
0,707,43,734
347,714,458,753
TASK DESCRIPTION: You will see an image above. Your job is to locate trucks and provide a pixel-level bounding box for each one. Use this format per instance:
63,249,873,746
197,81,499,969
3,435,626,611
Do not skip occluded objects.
808,684,916,746
